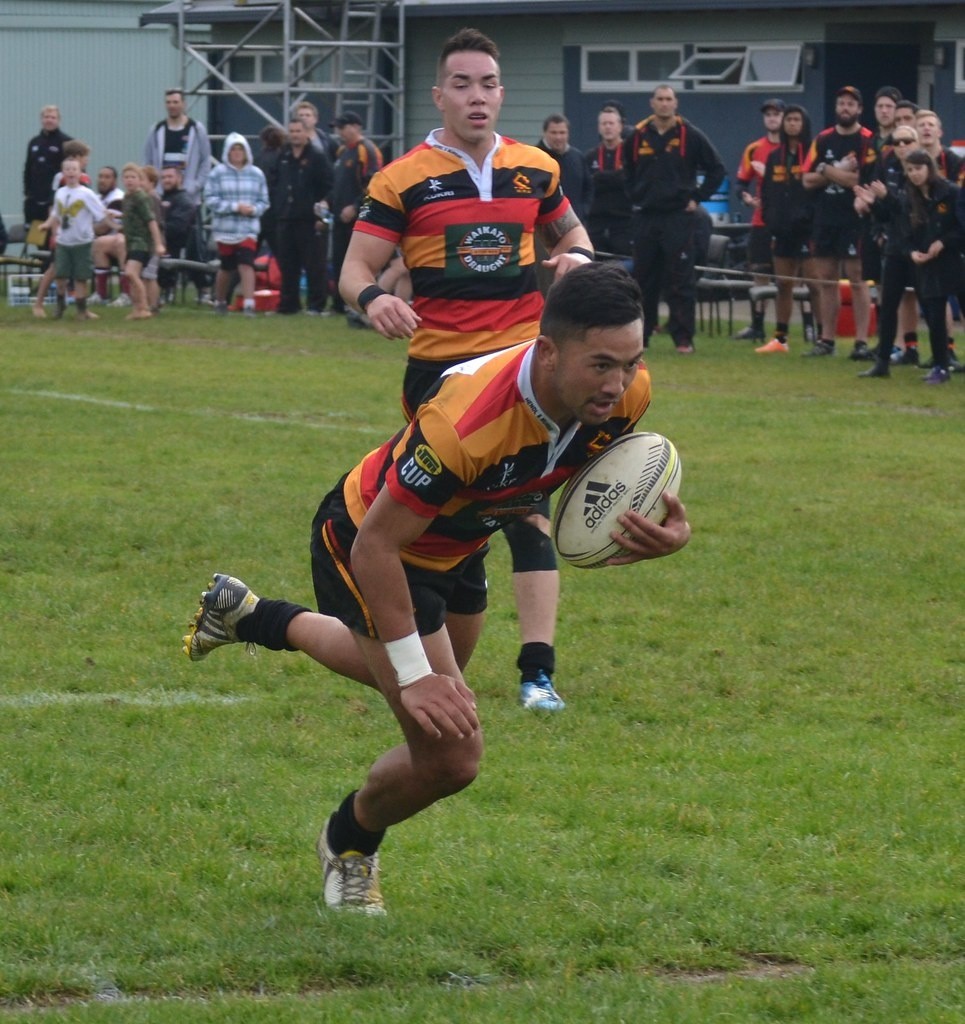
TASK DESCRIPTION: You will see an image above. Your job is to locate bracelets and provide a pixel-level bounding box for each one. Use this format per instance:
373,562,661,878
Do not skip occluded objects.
568,246,593,261
358,284,386,310
383,630,433,686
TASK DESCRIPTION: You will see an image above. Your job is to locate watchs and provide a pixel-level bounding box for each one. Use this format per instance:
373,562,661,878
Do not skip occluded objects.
816,162,826,174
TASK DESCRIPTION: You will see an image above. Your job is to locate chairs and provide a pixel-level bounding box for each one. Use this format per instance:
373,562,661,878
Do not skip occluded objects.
691,236,811,337
1,220,220,306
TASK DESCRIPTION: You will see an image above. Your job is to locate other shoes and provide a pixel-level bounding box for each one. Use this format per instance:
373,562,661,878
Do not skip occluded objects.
735,328,765,339
755,339,790,353
858,357,889,377
801,340,835,357
922,366,950,382
852,345,867,358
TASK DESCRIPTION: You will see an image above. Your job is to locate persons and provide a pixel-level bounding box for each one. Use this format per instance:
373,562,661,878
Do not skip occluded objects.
733,84,965,383
0,213,8,254
181,261,692,916
24,89,412,329
338,29,594,712
535,85,727,354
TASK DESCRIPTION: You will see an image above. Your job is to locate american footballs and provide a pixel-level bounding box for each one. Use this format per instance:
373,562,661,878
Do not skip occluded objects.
552,430,682,571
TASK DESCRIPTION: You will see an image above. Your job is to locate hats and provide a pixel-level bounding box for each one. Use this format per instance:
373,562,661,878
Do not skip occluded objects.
836,86,860,100
760,98,785,112
875,86,901,102
328,111,362,128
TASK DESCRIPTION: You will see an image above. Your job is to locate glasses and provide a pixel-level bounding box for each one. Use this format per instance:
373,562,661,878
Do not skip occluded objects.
893,138,915,146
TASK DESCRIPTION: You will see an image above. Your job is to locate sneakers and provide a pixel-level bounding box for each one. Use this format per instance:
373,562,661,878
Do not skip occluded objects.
317,817,386,916
522,669,565,710
182,573,258,661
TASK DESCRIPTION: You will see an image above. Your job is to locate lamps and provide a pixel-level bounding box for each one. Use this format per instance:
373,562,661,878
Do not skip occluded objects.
934,44,948,69
806,46,818,68
